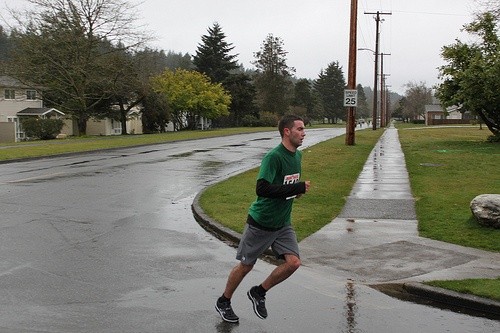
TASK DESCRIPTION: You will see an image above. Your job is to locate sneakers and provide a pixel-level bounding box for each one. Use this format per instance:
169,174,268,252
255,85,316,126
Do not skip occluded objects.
215,297,239,322
247,286,267,319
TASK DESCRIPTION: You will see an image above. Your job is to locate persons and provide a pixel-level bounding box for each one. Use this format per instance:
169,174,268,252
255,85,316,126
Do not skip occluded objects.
215,114,311,322
